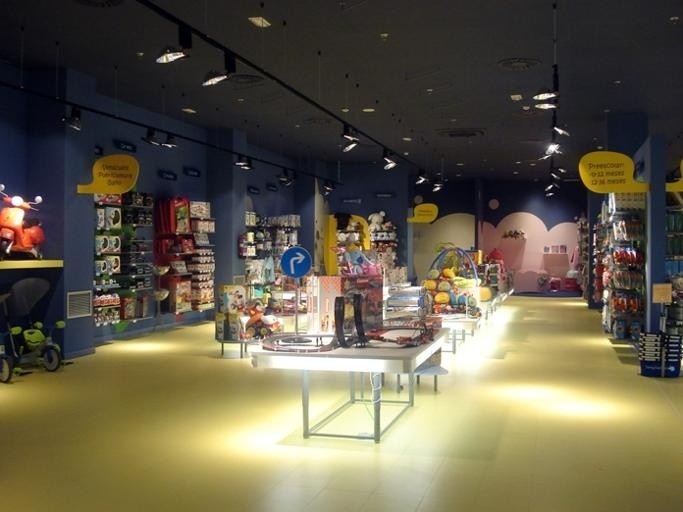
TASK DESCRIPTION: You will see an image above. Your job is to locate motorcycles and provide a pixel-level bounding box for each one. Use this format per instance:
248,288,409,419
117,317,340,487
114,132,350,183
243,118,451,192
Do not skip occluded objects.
0,181,51,260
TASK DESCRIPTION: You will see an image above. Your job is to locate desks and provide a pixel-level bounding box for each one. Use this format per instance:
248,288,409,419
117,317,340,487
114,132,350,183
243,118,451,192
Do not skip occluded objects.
250,327,450,444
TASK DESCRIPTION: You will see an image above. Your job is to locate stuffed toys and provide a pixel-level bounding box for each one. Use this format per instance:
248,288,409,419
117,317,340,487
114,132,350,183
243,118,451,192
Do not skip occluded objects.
238,208,482,353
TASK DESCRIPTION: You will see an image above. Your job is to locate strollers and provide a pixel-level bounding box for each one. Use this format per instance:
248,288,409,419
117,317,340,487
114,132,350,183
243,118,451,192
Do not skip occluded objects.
0,277,64,384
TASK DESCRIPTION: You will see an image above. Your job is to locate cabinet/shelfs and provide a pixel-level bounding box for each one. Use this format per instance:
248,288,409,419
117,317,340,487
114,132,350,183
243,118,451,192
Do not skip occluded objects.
573,133,681,379
